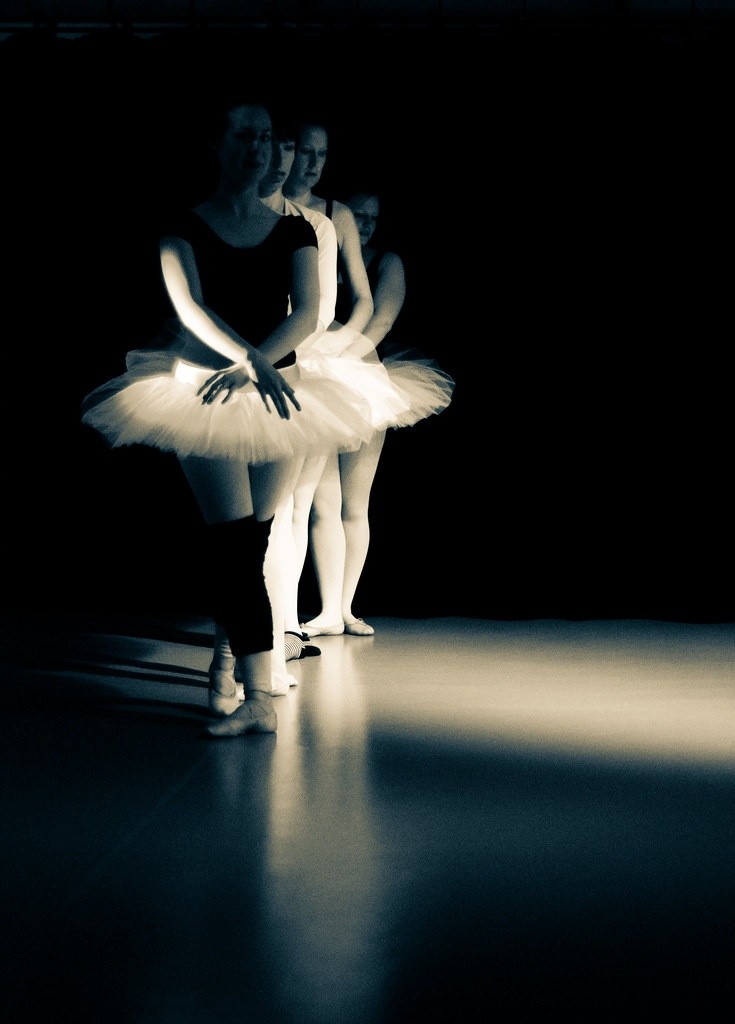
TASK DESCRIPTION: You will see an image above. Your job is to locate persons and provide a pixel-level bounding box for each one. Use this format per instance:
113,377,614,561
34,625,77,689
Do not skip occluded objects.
87,103,404,736
231,109,452,699
